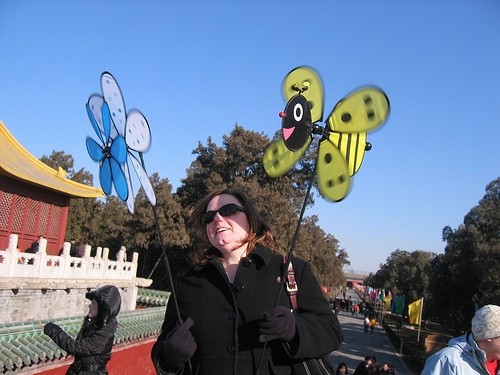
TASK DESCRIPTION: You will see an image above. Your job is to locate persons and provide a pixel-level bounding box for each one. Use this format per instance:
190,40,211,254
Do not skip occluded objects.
421,304,500,375
336,356,397,375
150,188,344,375
328,290,376,334
32,235,45,253
39,285,121,375
70,238,80,257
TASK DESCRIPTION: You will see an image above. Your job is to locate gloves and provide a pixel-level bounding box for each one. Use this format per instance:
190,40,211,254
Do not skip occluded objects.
44,323,53,334
158,319,196,369
258,307,296,342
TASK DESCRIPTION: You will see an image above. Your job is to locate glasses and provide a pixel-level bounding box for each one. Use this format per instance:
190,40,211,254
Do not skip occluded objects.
202,203,248,224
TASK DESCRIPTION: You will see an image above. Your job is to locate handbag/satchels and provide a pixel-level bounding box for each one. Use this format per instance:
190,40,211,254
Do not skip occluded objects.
292,358,336,375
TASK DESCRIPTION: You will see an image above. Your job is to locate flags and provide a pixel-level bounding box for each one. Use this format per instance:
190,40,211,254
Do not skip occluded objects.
355,284,405,316
408,298,421,324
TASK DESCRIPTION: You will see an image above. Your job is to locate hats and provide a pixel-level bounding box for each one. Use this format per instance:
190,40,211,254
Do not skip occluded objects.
471,304,500,341
